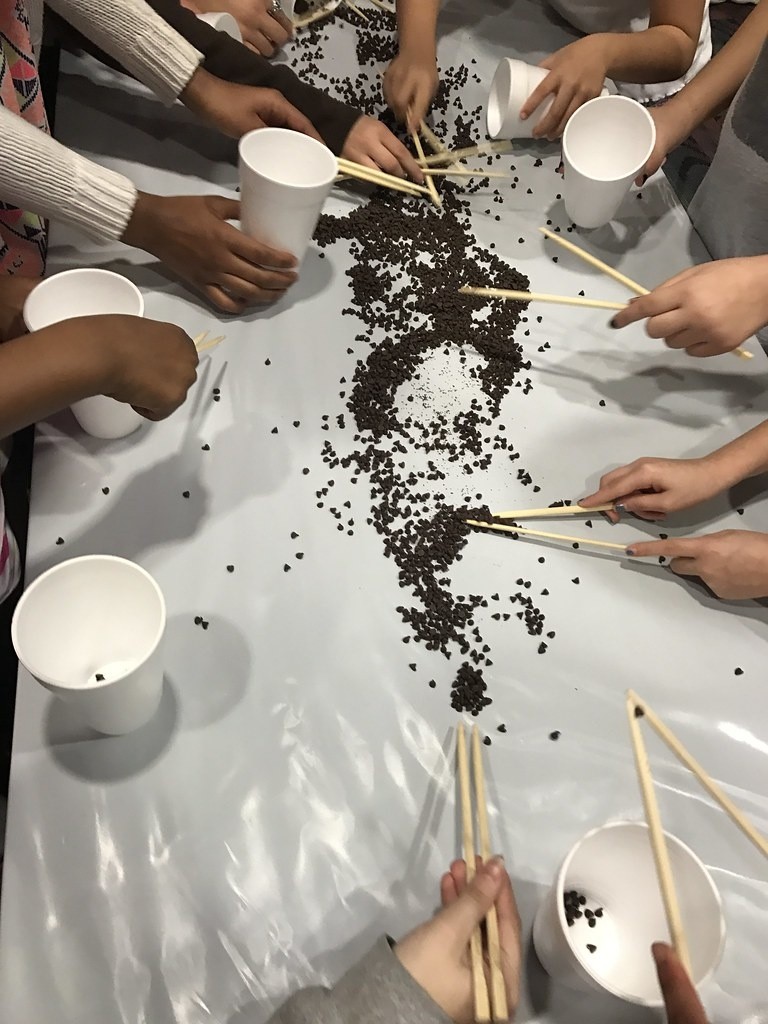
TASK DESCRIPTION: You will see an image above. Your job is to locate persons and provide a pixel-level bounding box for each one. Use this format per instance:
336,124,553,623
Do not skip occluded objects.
0,0,768,604
264,854,714,1023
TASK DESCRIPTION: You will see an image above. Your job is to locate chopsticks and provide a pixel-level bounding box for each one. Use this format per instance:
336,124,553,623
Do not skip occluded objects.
462,505,642,548
335,138,514,181
336,157,432,196
458,723,507,1023
372,68,471,208
627,688,768,975
190,330,226,355
458,226,757,360
342,0,394,21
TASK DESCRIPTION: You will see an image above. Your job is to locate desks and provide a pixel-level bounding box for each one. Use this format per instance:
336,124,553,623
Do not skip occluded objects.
0,0,768,1024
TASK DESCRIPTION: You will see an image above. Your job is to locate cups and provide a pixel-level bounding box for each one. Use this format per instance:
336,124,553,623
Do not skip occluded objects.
486,57,610,140
197,13,243,44
279,0,344,25
12,556,167,734
237,125,334,277
565,95,657,229
532,822,727,1005
24,269,147,440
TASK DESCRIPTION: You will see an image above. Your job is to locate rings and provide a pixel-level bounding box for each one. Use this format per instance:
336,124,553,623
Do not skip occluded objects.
270,0,283,13
665,333,679,347
